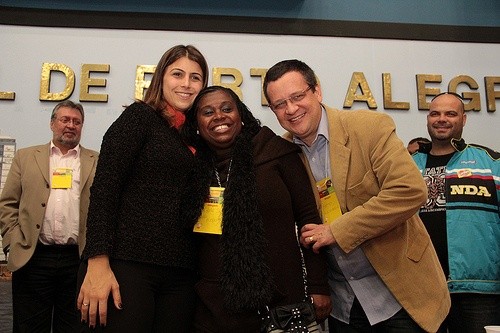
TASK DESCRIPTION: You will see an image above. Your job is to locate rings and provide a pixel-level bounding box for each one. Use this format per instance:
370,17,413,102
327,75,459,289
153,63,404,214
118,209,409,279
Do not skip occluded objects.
82,302,90,306
309,236,314,242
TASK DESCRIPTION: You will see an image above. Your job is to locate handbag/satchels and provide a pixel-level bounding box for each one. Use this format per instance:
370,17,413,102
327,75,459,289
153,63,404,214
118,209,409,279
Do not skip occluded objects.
259,296,325,333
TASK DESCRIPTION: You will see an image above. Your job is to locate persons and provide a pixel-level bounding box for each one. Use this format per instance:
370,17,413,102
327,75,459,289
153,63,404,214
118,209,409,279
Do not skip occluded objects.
410,93,500,333
191,85,332,333
77,45,214,333
1,99,100,333
264,59,452,333
407,138,431,155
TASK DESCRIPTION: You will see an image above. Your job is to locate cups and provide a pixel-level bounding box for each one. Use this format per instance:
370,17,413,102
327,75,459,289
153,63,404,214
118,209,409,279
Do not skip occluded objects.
484,326,500,333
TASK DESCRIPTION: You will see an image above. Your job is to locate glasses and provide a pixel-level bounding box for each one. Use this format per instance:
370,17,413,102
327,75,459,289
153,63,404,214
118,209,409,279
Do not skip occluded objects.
270,83,317,111
53,116,83,125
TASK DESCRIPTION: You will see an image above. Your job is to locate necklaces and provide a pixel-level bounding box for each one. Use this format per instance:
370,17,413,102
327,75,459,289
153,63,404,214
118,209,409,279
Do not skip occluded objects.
214,158,232,189
